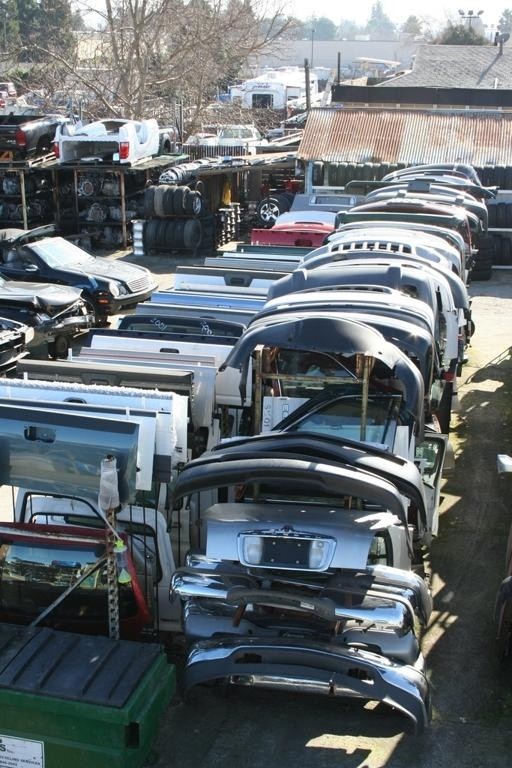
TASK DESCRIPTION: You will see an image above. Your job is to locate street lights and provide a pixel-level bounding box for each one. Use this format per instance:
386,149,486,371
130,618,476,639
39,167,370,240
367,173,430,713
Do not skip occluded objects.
458,9,484,28
310,27,316,69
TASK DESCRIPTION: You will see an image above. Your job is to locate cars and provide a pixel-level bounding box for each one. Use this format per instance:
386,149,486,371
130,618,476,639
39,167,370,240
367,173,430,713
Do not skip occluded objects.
185,124,269,158
0,224,159,361
